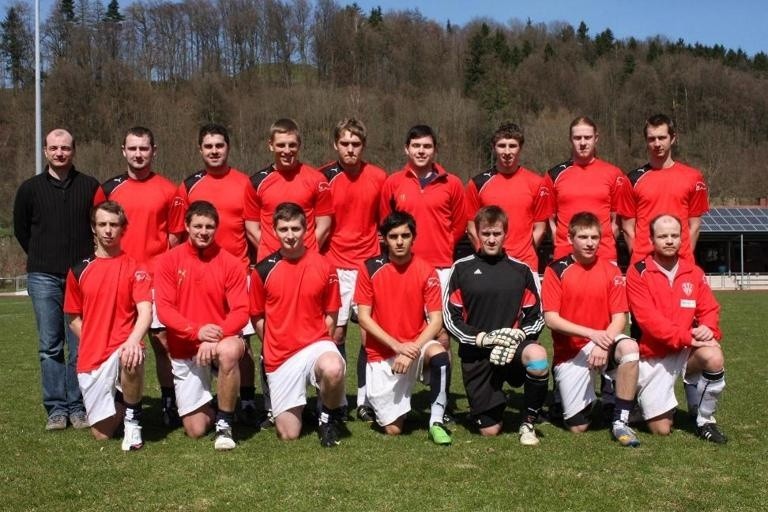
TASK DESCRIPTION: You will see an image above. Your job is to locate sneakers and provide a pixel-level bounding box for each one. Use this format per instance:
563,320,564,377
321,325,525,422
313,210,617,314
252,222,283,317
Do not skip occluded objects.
428,422,451,444
601,401,615,425
121,419,143,451
611,420,641,447
318,415,352,447
46,415,66,430
519,423,540,445
160,393,177,421
442,407,453,423
214,419,235,449
242,398,261,429
695,422,728,443
357,405,375,422
539,403,563,420
69,413,89,429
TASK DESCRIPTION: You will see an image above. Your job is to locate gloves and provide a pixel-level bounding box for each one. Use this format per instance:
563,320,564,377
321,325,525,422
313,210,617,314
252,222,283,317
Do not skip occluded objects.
477,328,524,349
490,331,526,365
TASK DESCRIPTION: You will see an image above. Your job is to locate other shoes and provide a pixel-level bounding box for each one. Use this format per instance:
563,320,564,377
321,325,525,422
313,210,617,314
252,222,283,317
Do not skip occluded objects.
265,408,274,425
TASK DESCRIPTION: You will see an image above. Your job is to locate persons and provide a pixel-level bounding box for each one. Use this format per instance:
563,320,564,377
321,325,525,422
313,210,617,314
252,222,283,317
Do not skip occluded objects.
461,123,552,412
626,215,728,444
541,115,636,424
167,123,262,421
376,124,469,424
244,118,333,430
443,206,549,446
541,211,641,446
94,125,178,423
153,200,250,450
351,212,452,445
621,114,708,415
14,128,99,430
310,117,387,423
62,199,154,451
248,202,346,447
